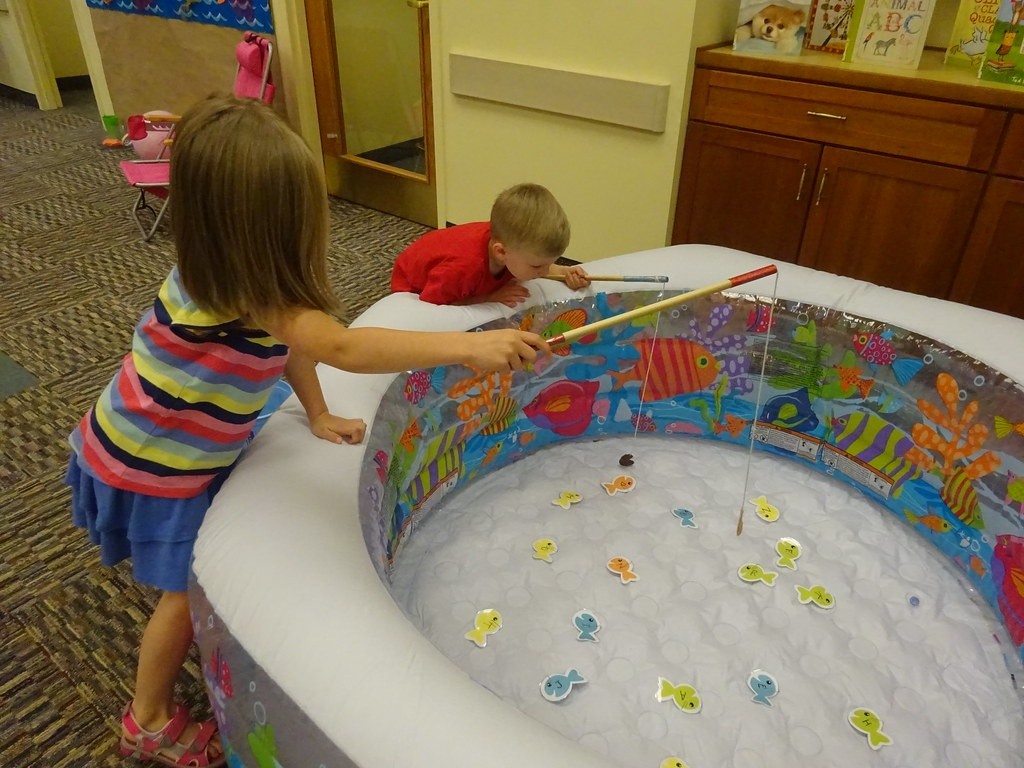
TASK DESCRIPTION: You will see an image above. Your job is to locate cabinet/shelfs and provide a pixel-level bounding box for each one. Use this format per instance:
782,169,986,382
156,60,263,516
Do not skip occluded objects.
671,52,1023,322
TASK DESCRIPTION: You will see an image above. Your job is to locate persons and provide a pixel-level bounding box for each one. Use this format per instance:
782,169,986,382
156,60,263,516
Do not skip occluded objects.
61,95,552,768
390,184,591,308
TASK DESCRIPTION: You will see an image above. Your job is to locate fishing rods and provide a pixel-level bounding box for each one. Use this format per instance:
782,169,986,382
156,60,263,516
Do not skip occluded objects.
517,262,779,359
544,273,670,284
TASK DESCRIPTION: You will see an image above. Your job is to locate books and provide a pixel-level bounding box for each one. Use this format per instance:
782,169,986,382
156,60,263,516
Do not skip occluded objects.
805,0,854,54
978,0,1024,87
851,0,937,70
943,0,999,71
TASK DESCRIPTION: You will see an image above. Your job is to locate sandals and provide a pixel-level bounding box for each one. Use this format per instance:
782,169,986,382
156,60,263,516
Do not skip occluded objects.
119,699,225,768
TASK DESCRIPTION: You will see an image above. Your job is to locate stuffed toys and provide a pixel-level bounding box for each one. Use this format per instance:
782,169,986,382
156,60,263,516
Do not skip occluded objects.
736,4,804,52
